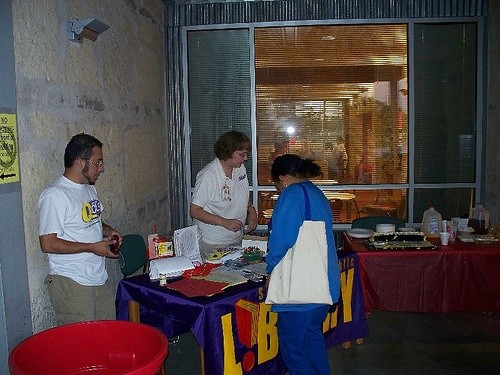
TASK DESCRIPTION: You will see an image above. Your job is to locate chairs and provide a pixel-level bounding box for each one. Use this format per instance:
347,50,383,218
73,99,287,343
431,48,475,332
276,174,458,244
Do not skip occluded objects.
351,216,406,232
119,234,147,277
354,189,403,221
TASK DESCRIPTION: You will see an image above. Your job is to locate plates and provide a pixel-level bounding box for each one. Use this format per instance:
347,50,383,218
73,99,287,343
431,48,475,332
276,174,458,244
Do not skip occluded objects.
375,223,395,233
347,227,374,238
397,228,415,232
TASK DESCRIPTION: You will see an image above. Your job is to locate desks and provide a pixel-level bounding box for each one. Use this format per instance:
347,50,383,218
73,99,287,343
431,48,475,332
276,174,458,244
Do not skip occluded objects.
324,192,356,224
342,232,500,313
114,251,369,375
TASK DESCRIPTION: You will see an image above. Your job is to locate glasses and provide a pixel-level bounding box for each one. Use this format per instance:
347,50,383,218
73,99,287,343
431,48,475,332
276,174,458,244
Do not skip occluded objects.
79,158,104,168
235,151,249,158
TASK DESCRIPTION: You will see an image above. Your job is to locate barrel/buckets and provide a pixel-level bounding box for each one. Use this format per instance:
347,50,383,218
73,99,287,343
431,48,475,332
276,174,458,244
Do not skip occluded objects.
468,205,487,227
472,203,490,235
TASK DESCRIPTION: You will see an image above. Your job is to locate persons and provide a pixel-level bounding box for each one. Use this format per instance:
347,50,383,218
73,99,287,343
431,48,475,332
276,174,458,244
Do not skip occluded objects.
191,129,257,261
38,132,123,326
264,154,341,375
266,136,348,174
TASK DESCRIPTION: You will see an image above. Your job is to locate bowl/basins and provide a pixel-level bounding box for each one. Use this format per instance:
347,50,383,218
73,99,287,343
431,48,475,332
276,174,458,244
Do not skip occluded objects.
405,223,422,230
8,320,167,375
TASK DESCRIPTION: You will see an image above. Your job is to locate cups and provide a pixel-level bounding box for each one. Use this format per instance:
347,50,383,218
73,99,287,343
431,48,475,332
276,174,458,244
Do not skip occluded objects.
441,232,449,245
451,217,474,235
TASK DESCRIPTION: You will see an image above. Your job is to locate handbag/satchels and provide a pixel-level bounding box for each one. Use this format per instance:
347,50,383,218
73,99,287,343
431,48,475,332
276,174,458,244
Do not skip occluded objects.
265,183,335,307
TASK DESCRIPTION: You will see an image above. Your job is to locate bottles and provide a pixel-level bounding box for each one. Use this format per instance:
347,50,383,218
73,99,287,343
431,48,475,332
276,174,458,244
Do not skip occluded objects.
442,220,455,242
241,246,264,264
422,207,442,238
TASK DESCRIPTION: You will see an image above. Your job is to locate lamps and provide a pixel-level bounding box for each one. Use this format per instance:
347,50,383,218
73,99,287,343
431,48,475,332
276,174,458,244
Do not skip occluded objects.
66,17,110,43
